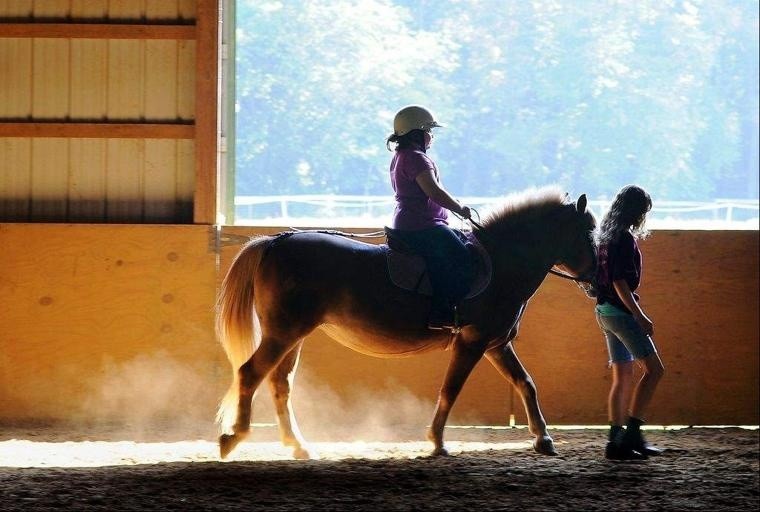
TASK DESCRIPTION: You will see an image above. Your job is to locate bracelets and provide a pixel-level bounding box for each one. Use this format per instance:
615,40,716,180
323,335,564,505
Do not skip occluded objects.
635,311,640,322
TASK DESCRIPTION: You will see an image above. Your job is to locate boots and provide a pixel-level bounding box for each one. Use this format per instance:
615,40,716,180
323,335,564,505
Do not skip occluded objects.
605,427,649,460
616,415,662,457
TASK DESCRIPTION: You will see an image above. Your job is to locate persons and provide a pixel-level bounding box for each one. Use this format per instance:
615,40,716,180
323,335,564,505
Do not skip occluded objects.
385,104,474,336
592,183,666,463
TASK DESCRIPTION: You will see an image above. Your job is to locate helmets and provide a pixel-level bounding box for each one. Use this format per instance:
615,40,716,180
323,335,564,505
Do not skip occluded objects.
394,106,441,136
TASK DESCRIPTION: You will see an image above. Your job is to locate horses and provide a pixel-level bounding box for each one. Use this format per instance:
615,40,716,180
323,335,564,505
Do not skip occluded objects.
211,190,609,462
